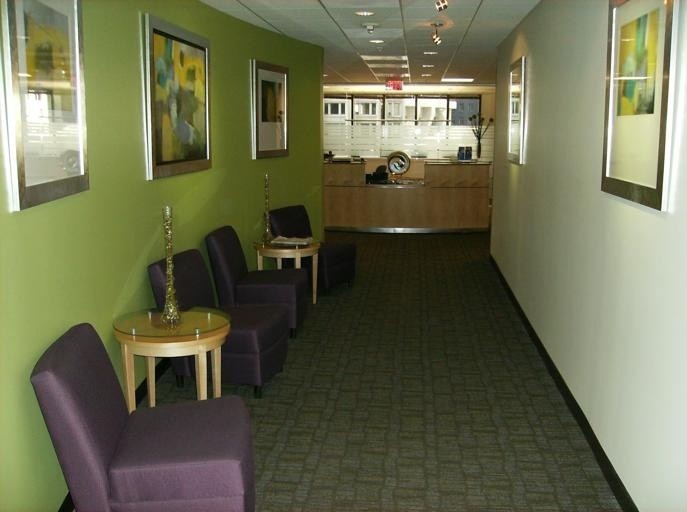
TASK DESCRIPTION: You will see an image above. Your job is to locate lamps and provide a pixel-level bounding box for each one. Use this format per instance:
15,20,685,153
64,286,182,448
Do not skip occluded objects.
29,320,255,510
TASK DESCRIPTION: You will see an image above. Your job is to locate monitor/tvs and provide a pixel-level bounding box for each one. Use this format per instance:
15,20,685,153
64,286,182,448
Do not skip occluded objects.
271,234,312,245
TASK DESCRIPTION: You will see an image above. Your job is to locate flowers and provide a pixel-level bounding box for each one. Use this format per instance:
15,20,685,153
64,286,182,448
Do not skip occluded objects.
467,112,493,136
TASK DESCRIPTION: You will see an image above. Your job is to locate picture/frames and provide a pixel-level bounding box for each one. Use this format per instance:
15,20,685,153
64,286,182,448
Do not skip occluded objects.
504,56,527,167
0,0,93,214
598,0,679,214
141,11,291,182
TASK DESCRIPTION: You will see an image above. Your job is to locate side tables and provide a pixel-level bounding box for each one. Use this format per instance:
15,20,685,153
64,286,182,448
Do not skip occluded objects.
254,237,320,304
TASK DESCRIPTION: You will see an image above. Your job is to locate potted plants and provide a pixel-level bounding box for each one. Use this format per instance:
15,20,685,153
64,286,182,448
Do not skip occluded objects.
504,56,527,167
141,11,291,182
0,0,93,214
598,0,679,214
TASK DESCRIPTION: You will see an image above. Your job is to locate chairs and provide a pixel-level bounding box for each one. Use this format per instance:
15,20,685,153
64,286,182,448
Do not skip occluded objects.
147,247,290,394
204,224,309,338
263,205,357,296
29,320,255,510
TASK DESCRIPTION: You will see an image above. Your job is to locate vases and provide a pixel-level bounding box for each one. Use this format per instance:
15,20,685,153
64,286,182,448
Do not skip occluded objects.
467,112,493,136
475,138,482,158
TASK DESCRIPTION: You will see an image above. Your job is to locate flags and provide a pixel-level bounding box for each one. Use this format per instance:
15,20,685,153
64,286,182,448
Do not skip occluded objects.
475,138,482,158
